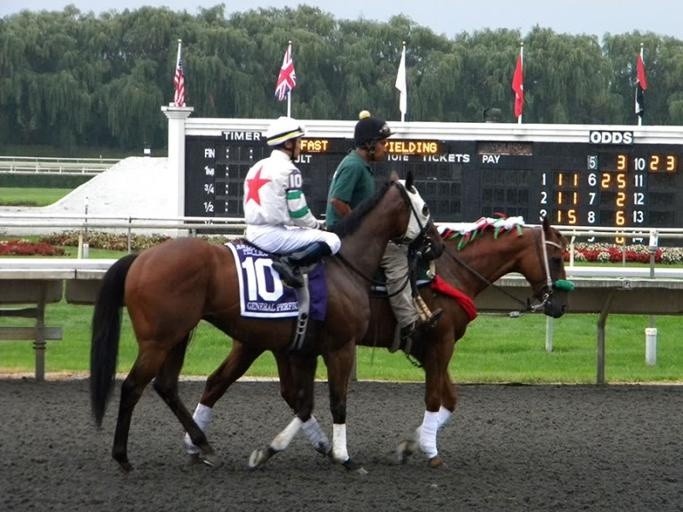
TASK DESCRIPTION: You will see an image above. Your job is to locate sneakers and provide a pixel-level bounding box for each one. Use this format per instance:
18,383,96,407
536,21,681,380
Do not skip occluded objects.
399,307,443,340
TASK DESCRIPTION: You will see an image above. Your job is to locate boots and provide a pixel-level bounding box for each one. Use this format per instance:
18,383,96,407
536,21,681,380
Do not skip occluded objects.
272,241,322,289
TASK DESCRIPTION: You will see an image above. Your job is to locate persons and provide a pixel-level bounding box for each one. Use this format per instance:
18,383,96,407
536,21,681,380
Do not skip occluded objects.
243,116,343,288
325,117,444,340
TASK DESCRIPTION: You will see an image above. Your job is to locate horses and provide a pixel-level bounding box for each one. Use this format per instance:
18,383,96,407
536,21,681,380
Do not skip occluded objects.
178,211,571,470
88,168,447,477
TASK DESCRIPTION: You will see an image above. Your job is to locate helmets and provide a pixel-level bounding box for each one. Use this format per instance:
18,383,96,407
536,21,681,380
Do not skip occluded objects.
265,116,304,146
354,117,397,143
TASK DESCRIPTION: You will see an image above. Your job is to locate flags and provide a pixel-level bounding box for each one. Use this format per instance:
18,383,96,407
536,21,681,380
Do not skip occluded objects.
634,56,647,117
274,45,296,101
174,44,186,107
395,45,408,114
512,47,525,117
